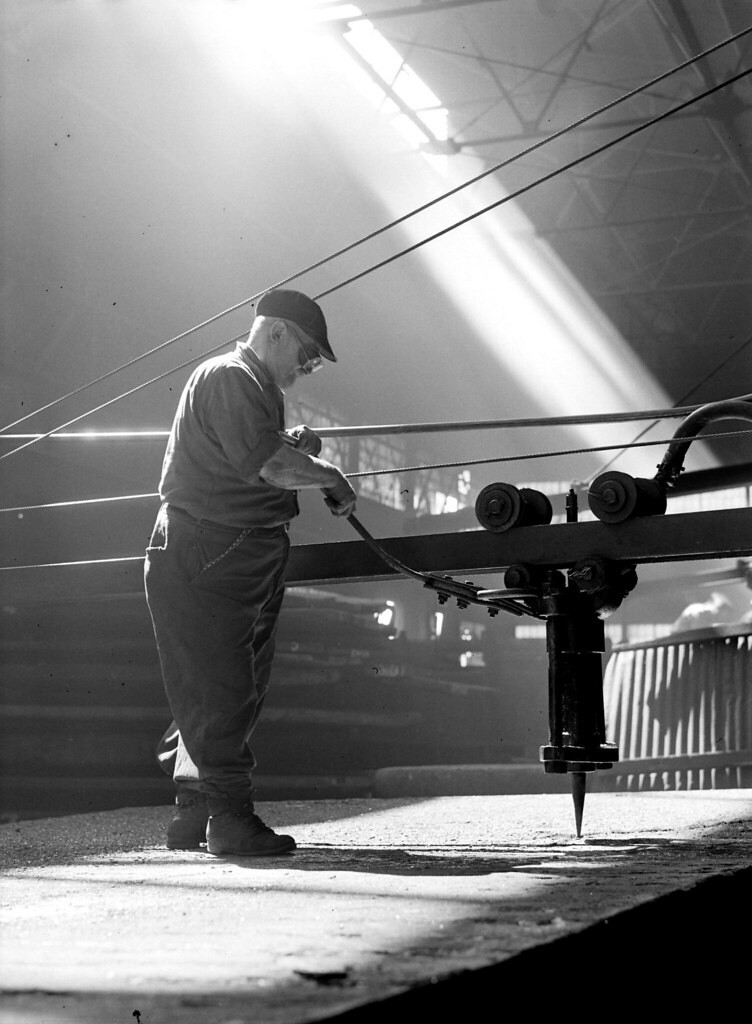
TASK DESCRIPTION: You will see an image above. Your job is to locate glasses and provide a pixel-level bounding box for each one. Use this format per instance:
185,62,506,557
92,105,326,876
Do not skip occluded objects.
287,325,324,375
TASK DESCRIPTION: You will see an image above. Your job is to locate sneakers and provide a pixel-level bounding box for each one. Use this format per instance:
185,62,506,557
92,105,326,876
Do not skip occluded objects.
166,806,211,849
206,812,296,855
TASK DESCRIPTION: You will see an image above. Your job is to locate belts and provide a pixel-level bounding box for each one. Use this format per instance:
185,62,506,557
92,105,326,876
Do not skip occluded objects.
165,504,291,534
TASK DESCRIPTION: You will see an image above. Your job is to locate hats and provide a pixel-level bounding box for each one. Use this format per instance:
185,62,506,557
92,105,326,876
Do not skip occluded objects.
256,289,337,362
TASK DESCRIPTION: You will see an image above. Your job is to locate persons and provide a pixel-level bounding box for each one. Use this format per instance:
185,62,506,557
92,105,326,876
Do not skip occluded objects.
143,288,358,856
